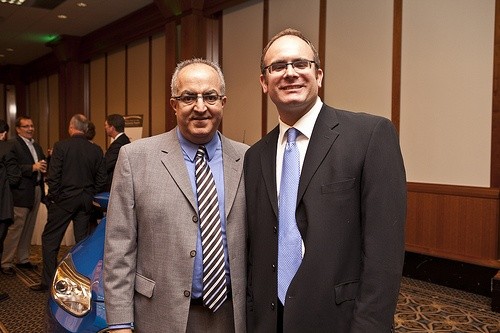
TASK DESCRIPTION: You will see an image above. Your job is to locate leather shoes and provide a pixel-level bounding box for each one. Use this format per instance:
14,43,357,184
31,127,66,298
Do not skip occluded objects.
30,284,49,291
2,267,16,277
15,262,37,271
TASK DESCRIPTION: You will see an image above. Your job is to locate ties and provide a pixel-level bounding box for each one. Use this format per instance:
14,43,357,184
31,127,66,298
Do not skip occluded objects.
277,128,302,307
195,145,229,314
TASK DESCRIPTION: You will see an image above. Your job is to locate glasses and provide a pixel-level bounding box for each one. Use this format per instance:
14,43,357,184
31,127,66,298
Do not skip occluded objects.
171,92,224,105
262,60,318,74
18,124,35,128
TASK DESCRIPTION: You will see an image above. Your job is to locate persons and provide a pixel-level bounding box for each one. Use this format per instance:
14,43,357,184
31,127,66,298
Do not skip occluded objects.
104,60,253,330
245,30,406,332
0,111,131,294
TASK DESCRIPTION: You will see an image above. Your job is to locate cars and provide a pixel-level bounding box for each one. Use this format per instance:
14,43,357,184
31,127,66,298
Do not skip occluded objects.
44,193,110,333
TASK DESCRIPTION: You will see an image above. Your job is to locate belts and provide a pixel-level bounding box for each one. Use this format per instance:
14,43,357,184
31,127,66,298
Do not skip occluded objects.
190,291,232,306
